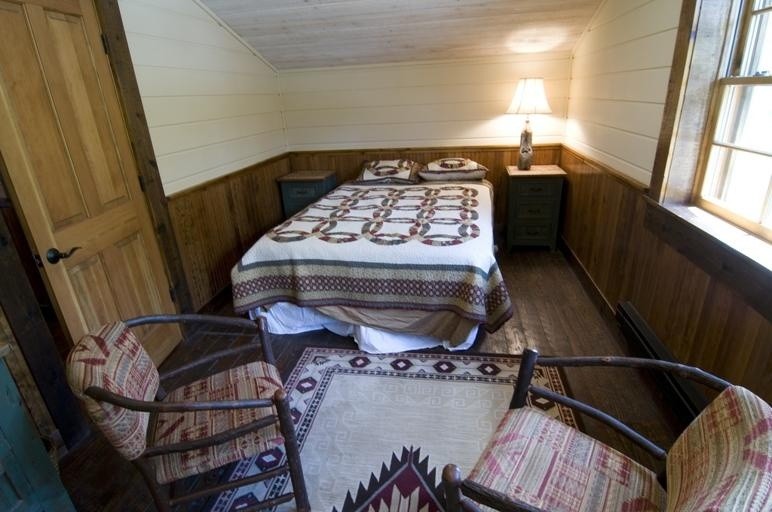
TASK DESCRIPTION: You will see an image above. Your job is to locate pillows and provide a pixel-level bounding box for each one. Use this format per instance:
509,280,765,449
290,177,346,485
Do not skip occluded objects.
351,159,489,184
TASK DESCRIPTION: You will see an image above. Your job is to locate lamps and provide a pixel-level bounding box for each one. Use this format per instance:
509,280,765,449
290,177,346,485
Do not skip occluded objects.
504,78,553,170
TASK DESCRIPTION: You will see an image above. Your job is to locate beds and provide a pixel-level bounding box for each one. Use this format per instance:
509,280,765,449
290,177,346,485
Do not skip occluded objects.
231,179,514,355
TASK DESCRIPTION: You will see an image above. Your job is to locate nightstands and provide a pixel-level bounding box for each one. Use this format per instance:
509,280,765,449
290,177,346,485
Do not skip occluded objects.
276,171,336,219
504,165,568,256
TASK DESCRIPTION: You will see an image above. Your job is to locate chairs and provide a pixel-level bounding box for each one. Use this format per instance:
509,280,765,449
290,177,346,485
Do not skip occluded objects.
64,314,311,512
443,348,772,512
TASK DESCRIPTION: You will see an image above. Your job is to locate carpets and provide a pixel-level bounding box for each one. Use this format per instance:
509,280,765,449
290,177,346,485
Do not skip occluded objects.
210,347,586,512
